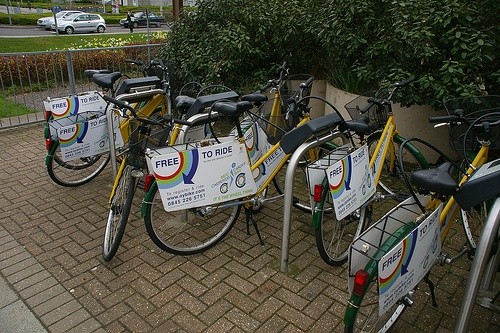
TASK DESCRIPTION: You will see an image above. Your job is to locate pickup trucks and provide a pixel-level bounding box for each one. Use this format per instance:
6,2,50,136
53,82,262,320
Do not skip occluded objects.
118,12,167,28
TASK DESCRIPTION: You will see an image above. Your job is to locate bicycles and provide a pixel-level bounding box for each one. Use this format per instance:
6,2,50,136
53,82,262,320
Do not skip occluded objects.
42,62,500,333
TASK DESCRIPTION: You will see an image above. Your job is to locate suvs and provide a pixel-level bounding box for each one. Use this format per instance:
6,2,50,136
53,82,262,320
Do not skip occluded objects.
37,10,107,34
